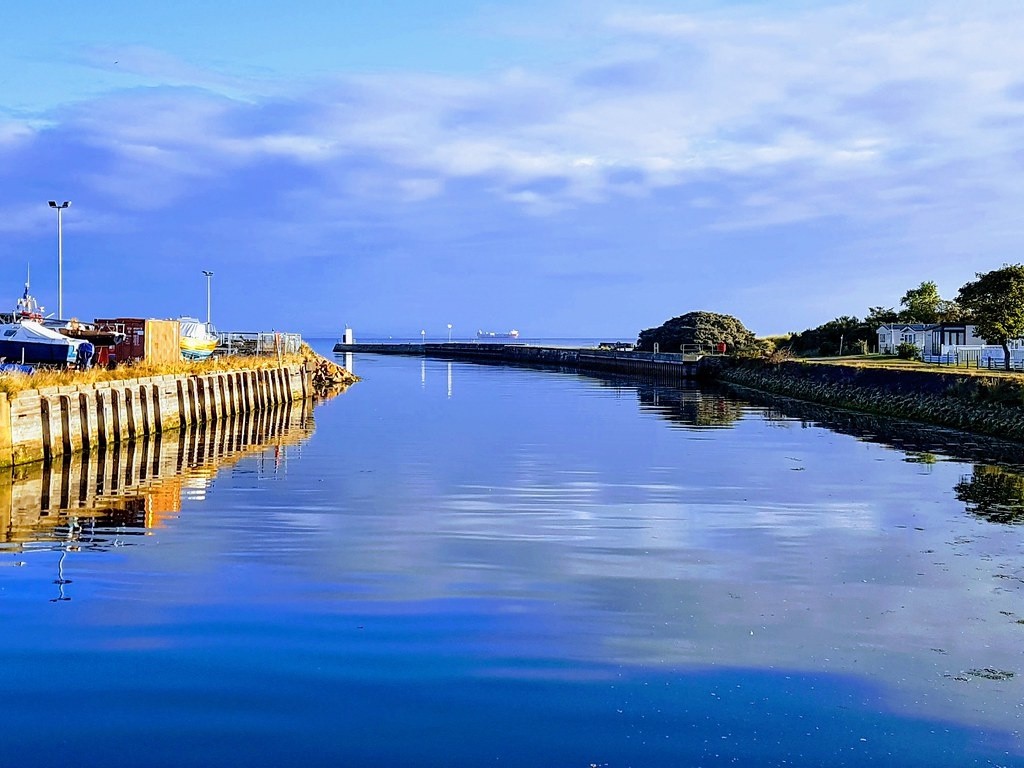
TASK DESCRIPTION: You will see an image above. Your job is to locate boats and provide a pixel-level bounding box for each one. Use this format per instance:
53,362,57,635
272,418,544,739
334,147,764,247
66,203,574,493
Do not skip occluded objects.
477,328,519,339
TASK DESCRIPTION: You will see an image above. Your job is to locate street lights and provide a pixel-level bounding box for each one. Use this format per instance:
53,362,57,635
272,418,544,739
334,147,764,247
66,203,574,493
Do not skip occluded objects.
201,270,214,334
447,324,452,343
420,330,426,342
48,199,72,318
